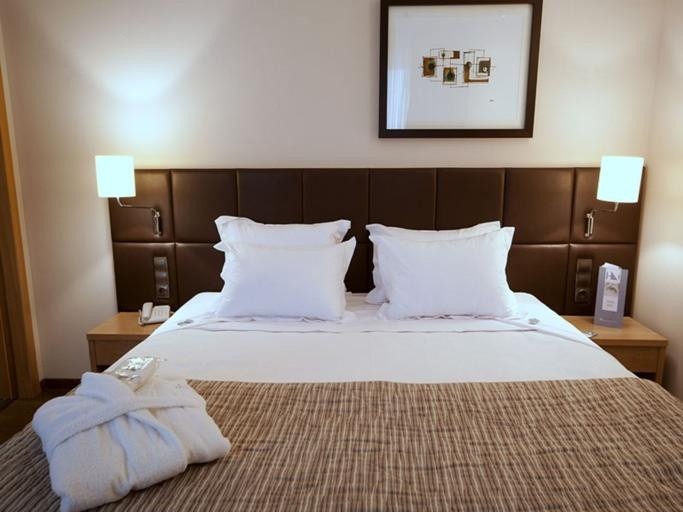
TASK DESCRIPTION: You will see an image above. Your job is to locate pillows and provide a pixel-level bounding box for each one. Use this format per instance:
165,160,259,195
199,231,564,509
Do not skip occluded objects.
211,235,357,323
214,214,352,246
368,226,526,323
364,220,502,305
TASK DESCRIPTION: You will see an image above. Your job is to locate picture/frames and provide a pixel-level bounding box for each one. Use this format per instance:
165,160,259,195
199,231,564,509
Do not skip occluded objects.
376,0,543,139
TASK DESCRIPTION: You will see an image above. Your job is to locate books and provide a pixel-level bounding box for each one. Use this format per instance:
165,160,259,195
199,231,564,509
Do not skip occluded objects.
593,262,629,328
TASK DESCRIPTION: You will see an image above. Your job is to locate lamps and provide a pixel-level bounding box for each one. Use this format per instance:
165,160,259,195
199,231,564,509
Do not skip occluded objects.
583,154,645,239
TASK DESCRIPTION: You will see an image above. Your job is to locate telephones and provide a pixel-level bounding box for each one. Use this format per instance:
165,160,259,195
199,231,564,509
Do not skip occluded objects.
142,302,170,324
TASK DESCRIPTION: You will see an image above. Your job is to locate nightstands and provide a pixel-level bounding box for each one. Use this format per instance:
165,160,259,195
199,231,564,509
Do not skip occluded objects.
87,310,174,374
562,313,670,387
93,154,162,237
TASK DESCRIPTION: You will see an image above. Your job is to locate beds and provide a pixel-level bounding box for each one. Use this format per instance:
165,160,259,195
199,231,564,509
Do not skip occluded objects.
0,289,683,512
